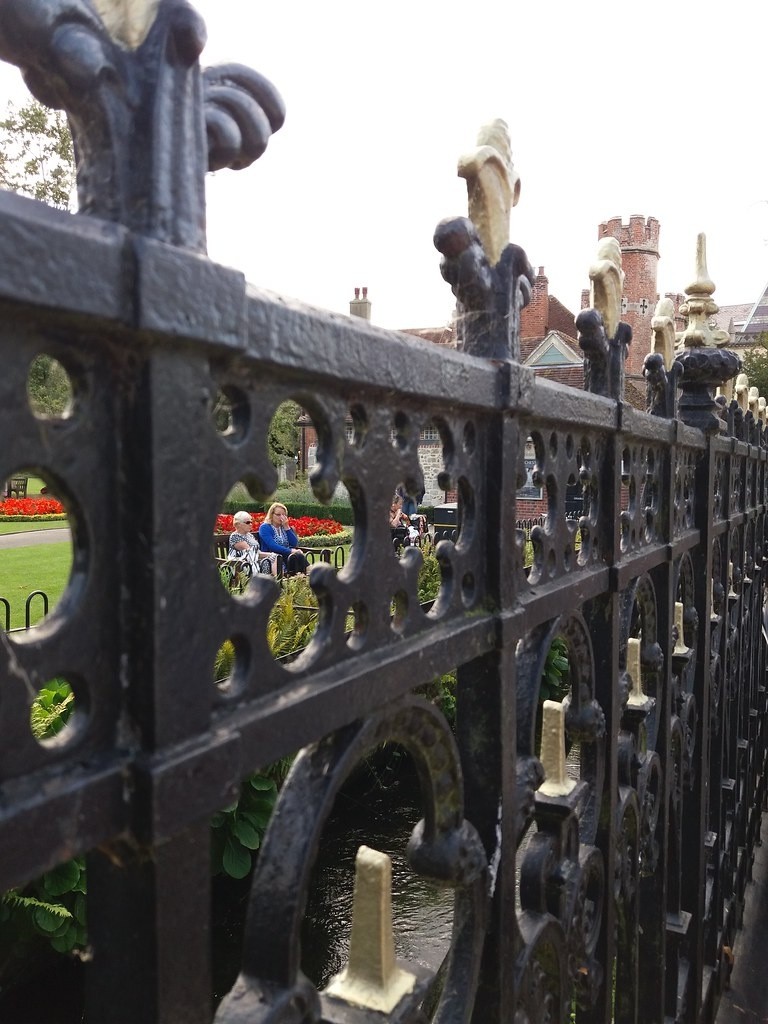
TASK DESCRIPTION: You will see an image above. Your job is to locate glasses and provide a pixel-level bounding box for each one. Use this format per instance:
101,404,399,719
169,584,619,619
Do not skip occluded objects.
242,521,253,524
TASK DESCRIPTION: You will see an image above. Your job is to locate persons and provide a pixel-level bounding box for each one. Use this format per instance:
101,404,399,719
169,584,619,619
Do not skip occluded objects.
259,502,310,576
389,494,419,547
229,510,284,576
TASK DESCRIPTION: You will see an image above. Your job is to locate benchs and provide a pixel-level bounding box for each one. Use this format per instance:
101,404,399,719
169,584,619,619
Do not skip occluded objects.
211,532,331,591
7,475,28,499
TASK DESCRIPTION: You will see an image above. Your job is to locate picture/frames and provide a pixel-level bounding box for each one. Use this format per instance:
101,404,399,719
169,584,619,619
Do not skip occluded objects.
514,436,543,501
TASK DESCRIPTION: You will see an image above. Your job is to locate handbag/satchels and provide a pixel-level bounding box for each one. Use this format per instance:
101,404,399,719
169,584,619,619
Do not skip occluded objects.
236,544,260,575
